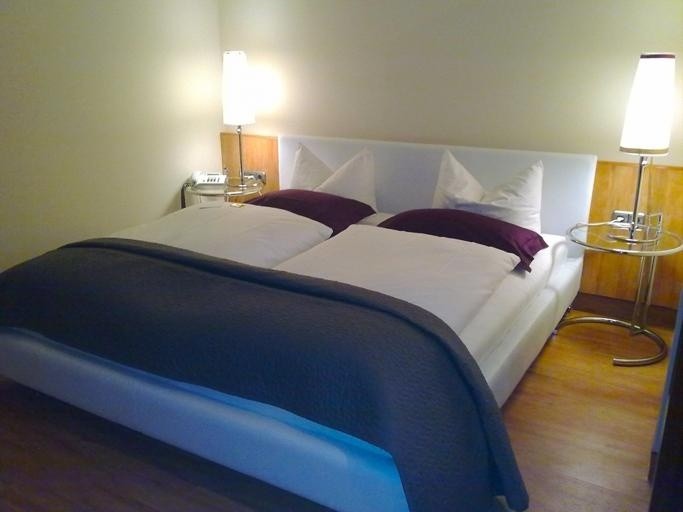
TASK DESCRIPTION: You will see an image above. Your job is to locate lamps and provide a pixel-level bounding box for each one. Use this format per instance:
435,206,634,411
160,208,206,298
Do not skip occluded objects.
221,48,257,189
616,53,674,244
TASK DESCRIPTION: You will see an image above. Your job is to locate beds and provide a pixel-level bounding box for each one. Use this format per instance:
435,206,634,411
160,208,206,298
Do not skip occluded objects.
0,134,598,512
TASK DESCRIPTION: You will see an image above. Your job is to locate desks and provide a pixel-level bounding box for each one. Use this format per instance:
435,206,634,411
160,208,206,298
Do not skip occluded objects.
185,177,261,203
551,221,683,367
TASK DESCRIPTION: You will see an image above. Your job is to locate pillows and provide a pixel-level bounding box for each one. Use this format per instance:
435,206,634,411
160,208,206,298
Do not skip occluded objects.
242,188,376,236
380,207,548,273
289,143,378,212
430,148,545,237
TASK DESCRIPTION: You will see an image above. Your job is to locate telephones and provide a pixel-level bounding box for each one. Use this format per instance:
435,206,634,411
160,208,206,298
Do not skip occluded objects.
192,170,227,185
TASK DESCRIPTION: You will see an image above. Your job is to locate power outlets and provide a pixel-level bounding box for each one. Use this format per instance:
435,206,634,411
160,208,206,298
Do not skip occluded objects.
613,210,644,230
241,170,266,185
